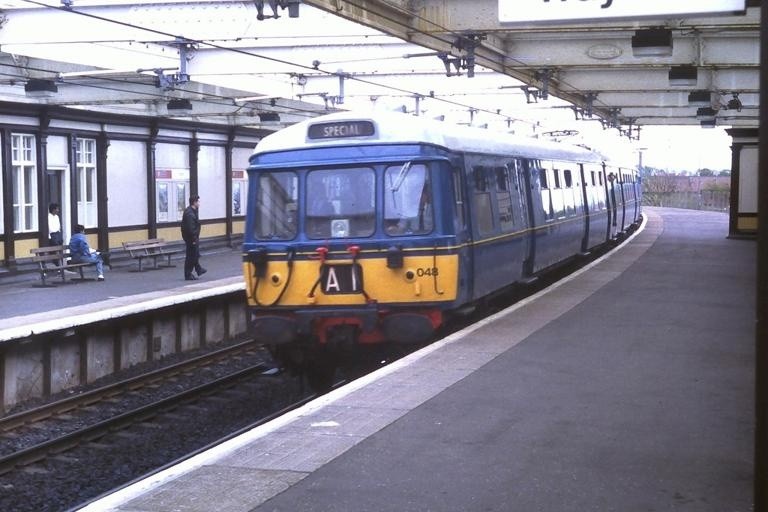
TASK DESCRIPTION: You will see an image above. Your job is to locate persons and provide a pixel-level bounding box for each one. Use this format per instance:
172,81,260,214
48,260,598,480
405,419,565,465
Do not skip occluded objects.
397,192,433,233
181,195,207,280
48,204,62,266
69,225,104,281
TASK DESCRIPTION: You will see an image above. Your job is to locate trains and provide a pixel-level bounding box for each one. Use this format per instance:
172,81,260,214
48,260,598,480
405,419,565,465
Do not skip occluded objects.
240,110,642,395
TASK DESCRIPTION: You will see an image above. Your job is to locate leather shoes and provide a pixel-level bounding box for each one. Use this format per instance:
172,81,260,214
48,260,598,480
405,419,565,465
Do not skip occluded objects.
197,269,207,276
184,275,198,280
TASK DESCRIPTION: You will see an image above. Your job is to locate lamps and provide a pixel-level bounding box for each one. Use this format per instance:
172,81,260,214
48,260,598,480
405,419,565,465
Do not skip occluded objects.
24,79,59,99
688,91,718,129
258,113,281,126
167,97,194,115
668,65,697,87
630,29,675,58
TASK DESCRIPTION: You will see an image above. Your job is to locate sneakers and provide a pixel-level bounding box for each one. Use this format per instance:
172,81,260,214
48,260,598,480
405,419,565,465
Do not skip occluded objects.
99,252,111,258
98,275,104,281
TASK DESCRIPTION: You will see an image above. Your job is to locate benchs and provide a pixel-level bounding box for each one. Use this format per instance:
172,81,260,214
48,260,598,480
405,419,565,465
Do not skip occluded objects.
122,238,177,272
30,245,98,287
